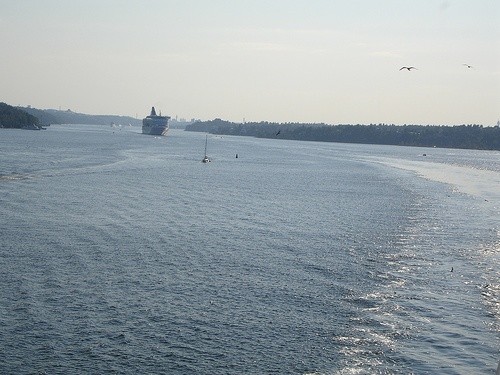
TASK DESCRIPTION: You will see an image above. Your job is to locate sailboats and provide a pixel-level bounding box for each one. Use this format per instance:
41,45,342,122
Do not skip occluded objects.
201,135,212,163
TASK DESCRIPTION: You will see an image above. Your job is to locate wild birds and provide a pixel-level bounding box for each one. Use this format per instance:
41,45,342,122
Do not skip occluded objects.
462,64,472,68
399,66,418,71
276,131,280,136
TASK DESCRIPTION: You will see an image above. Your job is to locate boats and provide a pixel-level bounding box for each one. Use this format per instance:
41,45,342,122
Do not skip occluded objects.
142,106,171,135
